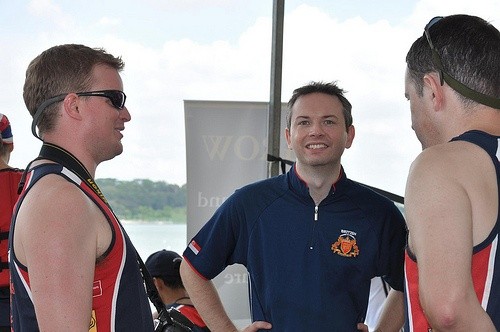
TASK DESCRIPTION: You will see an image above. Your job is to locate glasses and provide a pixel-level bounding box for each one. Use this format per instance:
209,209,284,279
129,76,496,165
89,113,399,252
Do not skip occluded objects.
425,15,444,70
74,90,127,109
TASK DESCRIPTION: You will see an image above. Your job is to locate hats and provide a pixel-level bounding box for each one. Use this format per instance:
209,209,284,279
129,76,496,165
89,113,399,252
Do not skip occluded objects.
1,113,14,145
144,248,182,277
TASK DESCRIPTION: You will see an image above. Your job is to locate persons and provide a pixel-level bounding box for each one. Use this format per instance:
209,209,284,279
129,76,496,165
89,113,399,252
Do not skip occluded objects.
0,113,26,332
145,248,211,332
403,14,500,332
179,81,408,332
9,43,156,332
